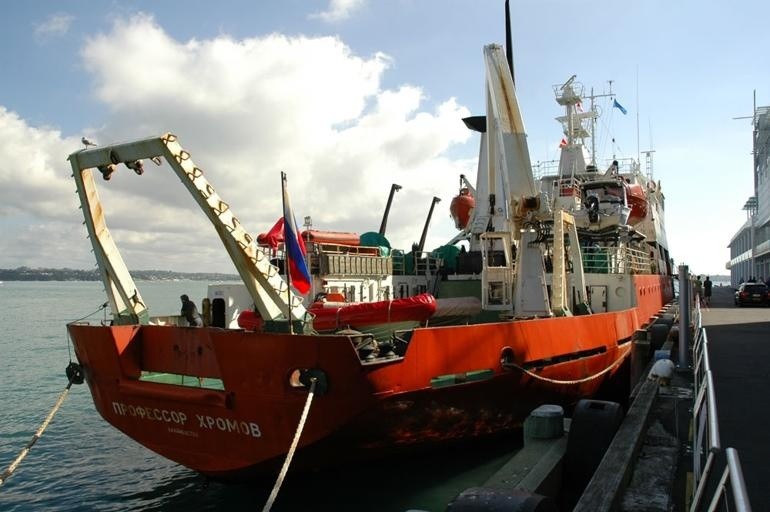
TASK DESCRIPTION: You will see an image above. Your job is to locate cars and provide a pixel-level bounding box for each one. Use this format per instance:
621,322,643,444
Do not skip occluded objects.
734,283,770,308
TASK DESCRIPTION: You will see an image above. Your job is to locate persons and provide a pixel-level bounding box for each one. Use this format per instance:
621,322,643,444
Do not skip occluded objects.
703,276,712,305
180,294,204,327
695,276,702,302
739,274,769,286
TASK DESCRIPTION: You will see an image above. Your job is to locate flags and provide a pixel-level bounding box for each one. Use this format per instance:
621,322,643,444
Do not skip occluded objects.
559,138,567,148
282,184,311,295
577,103,584,114
613,98,627,115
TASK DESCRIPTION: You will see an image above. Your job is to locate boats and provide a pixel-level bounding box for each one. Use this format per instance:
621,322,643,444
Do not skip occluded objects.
66,0,675,472
